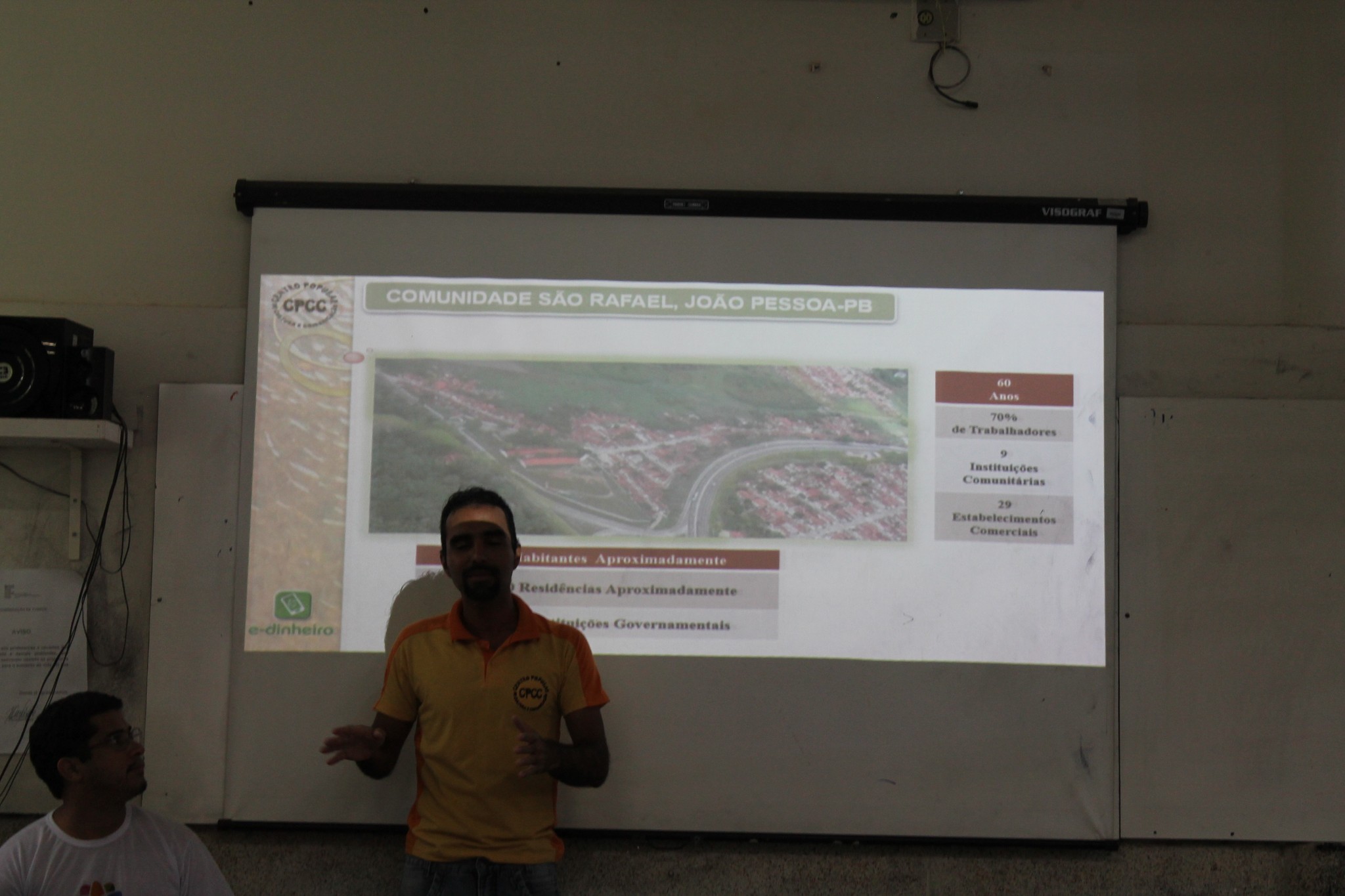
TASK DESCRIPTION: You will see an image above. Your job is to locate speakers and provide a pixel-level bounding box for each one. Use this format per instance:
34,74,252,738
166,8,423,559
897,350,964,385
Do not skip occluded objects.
1,315,115,420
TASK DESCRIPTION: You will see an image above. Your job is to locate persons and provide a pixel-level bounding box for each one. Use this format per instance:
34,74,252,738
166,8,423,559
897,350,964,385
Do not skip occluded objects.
0,689,240,895
321,486,612,896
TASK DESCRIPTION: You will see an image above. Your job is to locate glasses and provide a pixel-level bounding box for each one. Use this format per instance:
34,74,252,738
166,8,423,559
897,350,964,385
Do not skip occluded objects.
83,728,143,756
